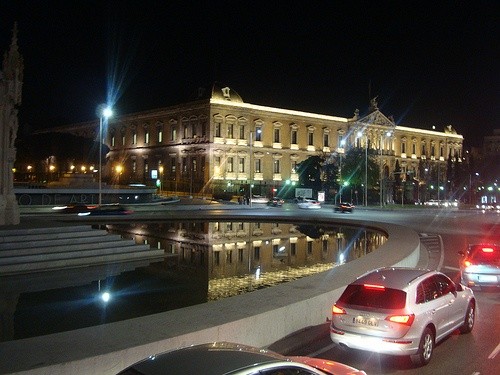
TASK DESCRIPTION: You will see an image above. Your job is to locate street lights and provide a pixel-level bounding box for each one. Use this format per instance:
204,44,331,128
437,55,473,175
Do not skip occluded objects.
250,183,254,208
99,106,112,204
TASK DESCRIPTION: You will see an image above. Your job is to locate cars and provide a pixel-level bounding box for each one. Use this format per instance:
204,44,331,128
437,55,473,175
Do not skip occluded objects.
297,198,321,209
457,244,500,287
330,267,477,366
333,202,353,213
111,341,368,375
